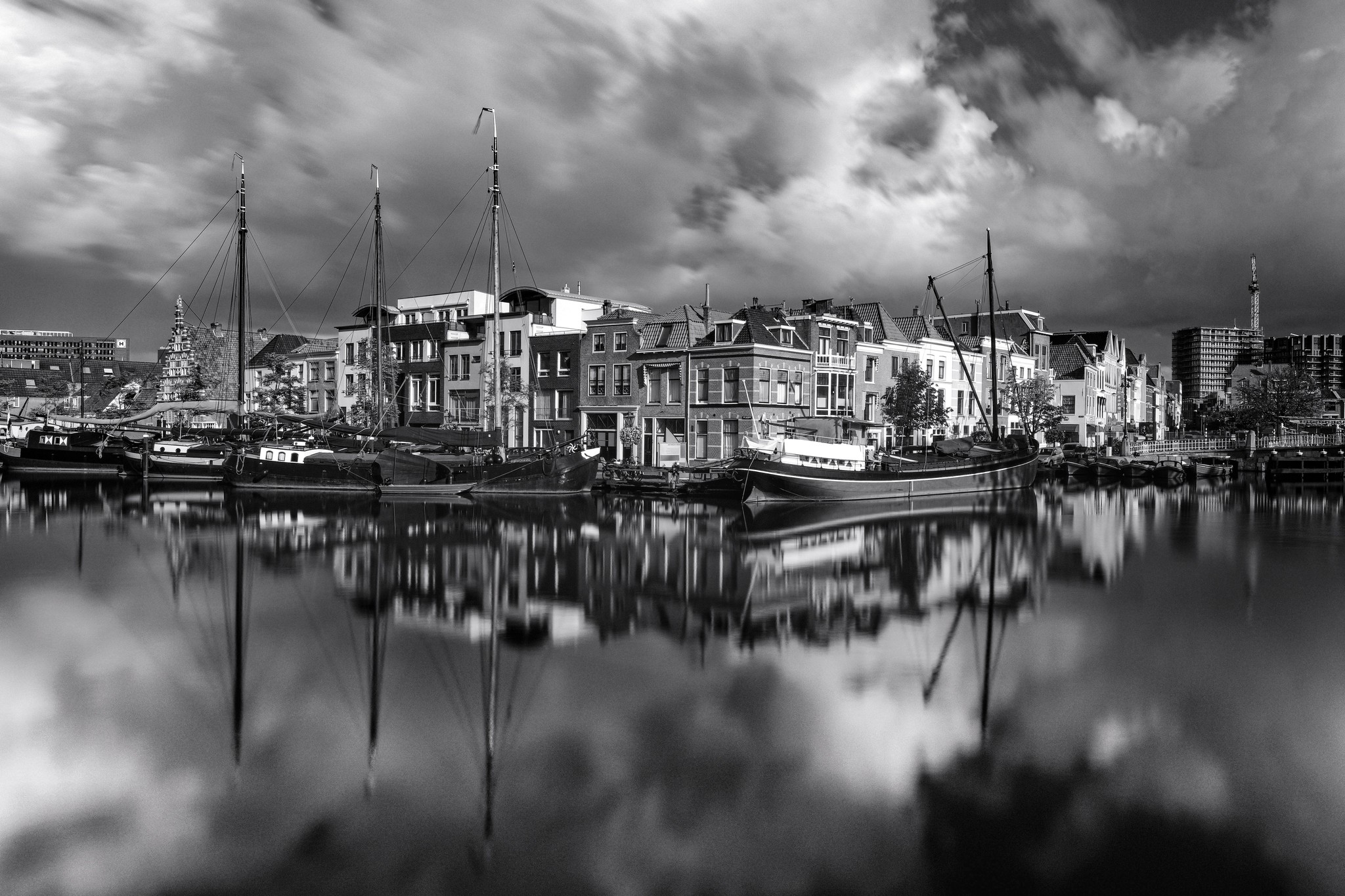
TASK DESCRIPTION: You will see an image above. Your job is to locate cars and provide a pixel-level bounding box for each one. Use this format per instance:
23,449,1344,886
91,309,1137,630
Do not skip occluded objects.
1037,447,1065,466
1062,443,1082,458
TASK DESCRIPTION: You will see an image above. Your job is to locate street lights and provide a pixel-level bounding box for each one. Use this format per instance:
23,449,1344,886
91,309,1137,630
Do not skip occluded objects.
1193,410,1202,435
1182,417,1187,435
1120,368,1133,456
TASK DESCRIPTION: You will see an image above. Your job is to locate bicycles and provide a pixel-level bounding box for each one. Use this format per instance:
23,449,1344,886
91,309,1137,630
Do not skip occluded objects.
1086,439,1122,459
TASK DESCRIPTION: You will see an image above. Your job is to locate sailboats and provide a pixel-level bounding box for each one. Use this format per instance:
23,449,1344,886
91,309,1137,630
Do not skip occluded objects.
0,103,1046,526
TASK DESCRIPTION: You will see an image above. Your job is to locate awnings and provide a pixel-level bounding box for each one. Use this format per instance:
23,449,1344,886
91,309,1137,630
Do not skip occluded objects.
1105,424,1123,433
1086,425,1104,432
1057,424,1079,433
841,417,890,428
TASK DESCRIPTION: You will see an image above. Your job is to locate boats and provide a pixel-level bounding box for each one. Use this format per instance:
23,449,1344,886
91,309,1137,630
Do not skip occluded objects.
1058,458,1089,475
1189,455,1233,477
1088,458,1121,476
1121,460,1157,480
1153,454,1186,480
